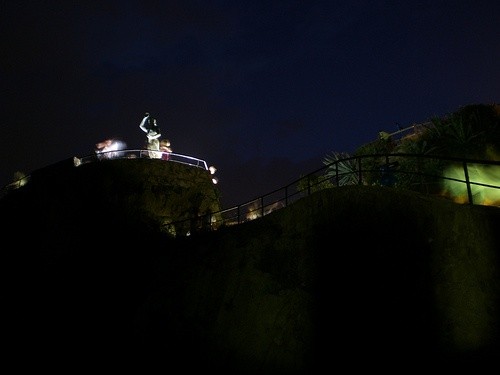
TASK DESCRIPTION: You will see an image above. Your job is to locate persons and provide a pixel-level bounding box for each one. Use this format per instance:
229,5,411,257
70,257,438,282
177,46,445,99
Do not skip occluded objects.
139,112,162,159
